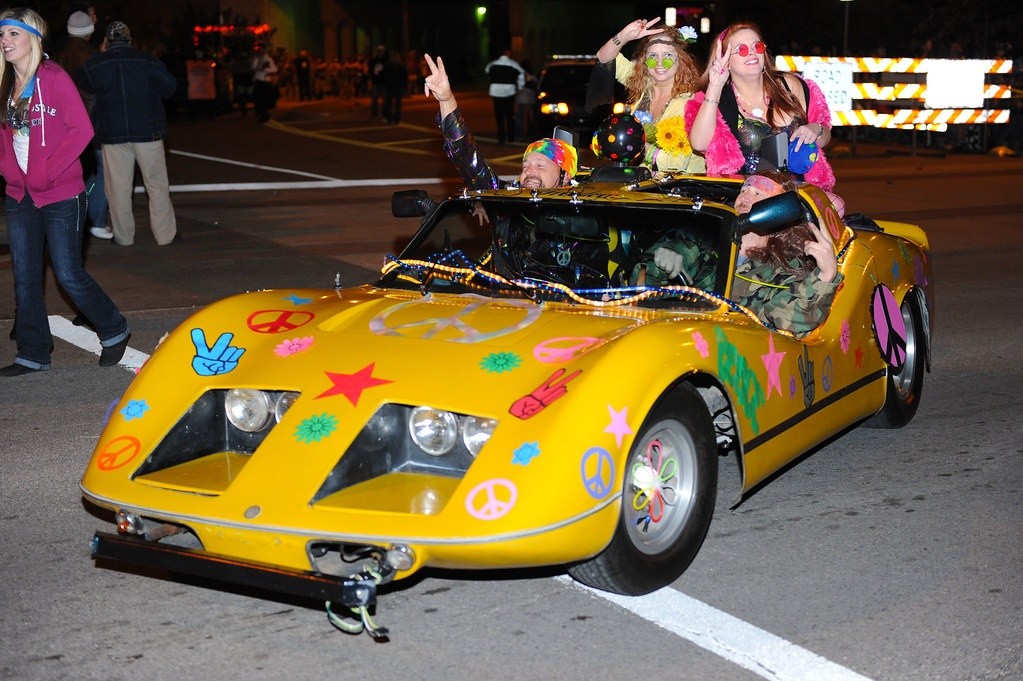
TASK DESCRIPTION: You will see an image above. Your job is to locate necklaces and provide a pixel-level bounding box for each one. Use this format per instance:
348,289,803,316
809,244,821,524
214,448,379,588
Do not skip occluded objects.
734,87,763,118
647,96,673,124
7,87,32,130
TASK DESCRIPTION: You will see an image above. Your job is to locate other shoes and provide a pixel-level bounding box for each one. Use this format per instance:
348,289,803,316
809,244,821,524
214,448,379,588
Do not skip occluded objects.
89,226,113,239
0,362,37,376
98,330,132,367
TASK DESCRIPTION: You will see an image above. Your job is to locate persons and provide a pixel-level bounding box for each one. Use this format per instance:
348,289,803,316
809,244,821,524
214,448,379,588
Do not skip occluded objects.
368,44,408,124
285,50,367,100
50,4,112,240
596,16,706,173
683,26,845,218
0,9,133,377
629,169,845,333
486,44,538,143
249,44,278,121
72,20,179,246
423,53,578,281
785,37,1023,154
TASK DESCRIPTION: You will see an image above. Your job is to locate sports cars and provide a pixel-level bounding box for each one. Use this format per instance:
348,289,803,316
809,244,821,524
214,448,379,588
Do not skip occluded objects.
83,172,941,639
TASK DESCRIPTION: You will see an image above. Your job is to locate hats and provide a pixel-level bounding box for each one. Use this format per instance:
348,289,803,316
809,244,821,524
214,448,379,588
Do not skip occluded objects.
106,21,130,41
66,11,95,37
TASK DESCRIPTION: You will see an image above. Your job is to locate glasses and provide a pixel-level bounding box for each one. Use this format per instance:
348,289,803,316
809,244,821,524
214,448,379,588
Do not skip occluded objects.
730,41,766,57
644,55,679,69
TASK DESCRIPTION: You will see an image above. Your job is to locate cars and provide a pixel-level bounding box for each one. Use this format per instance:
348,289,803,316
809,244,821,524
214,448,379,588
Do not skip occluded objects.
533,55,618,142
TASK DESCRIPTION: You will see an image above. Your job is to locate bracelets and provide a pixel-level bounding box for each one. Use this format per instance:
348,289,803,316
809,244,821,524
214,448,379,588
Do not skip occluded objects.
613,34,621,46
704,96,719,103
816,122,823,140
644,144,659,165
